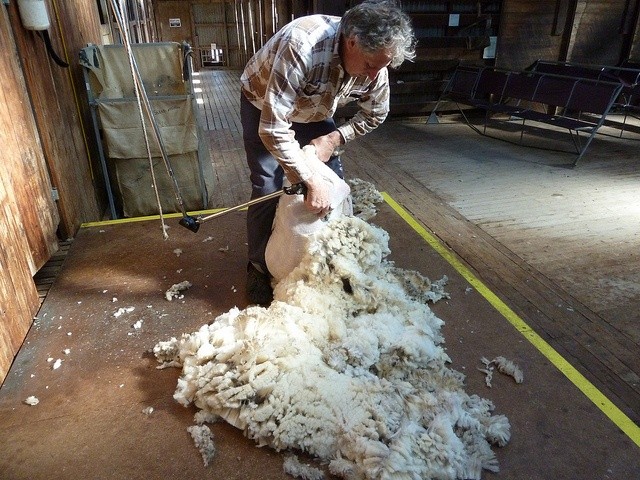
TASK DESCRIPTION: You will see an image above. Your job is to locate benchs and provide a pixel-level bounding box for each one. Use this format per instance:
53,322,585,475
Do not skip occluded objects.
509,59,640,139
426,62,624,172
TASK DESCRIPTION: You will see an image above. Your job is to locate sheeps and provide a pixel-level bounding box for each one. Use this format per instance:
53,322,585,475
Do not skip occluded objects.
265,145,353,282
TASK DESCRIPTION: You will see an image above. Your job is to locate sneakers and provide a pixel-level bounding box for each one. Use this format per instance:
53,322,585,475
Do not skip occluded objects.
247,262,274,309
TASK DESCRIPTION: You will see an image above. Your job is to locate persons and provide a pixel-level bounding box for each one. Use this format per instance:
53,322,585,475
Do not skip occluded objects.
240,1,419,308
